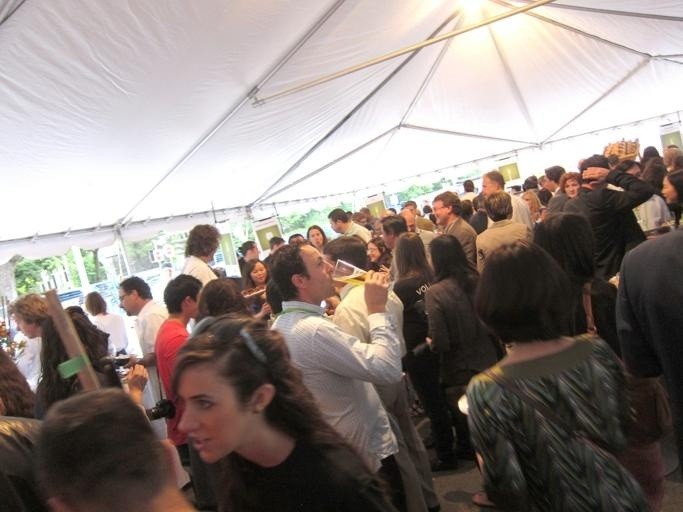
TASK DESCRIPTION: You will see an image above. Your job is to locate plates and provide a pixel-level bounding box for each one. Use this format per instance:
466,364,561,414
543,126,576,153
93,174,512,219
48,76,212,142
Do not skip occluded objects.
245,287,266,300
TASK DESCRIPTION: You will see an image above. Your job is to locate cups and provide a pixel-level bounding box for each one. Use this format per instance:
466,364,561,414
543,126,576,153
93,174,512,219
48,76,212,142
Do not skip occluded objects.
331,259,367,288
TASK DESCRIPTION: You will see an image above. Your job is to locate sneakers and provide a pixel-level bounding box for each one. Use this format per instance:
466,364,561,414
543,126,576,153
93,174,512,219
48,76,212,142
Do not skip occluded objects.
472,492,495,506
430,457,457,471
453,449,473,460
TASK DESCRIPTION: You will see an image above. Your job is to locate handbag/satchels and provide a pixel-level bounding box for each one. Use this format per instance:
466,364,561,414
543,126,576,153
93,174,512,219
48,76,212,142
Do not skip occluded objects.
622,376,674,447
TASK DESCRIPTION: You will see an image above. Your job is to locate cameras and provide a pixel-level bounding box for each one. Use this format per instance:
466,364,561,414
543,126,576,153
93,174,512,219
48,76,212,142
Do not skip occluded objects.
145,400,175,421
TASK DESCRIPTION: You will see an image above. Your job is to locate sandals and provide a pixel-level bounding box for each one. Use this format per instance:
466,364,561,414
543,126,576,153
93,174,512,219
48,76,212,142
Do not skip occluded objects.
410,401,426,417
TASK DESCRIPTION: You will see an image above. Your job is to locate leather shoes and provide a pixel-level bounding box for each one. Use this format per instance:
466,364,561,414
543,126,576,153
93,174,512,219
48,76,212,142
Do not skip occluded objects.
422,433,433,448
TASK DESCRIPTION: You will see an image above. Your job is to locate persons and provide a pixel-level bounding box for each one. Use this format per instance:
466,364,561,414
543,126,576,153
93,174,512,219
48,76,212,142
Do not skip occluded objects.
1,145,682,512
